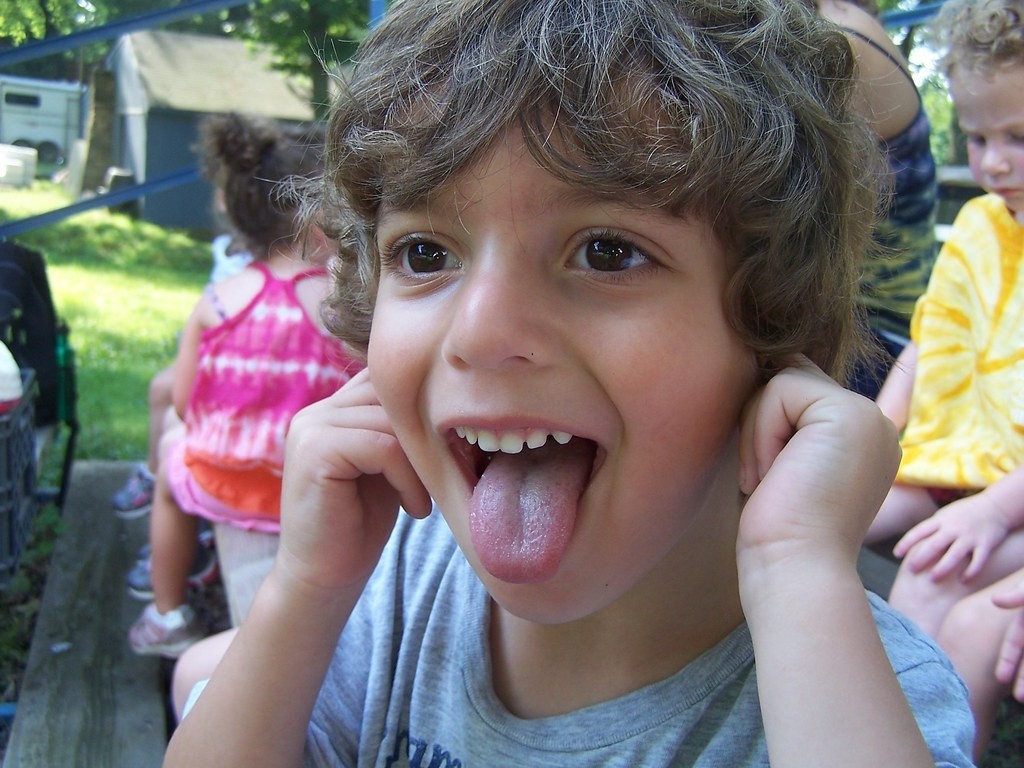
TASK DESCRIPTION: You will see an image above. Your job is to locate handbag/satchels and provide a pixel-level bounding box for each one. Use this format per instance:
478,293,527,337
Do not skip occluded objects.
0,241,72,425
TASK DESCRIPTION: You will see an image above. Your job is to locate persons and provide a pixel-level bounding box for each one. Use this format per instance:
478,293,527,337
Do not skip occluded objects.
154,0,984,768
816,1,938,405
101,114,370,661
857,1,1024,652
927,568,1024,768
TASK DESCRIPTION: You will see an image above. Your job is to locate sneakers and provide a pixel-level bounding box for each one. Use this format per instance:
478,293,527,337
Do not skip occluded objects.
140,531,214,556
112,465,155,519
126,555,220,600
129,605,199,658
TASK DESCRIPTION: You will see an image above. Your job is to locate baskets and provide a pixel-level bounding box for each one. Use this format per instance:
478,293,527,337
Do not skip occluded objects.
0,368,39,588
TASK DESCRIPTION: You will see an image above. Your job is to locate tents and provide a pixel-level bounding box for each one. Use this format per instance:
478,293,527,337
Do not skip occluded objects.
60,28,366,248
